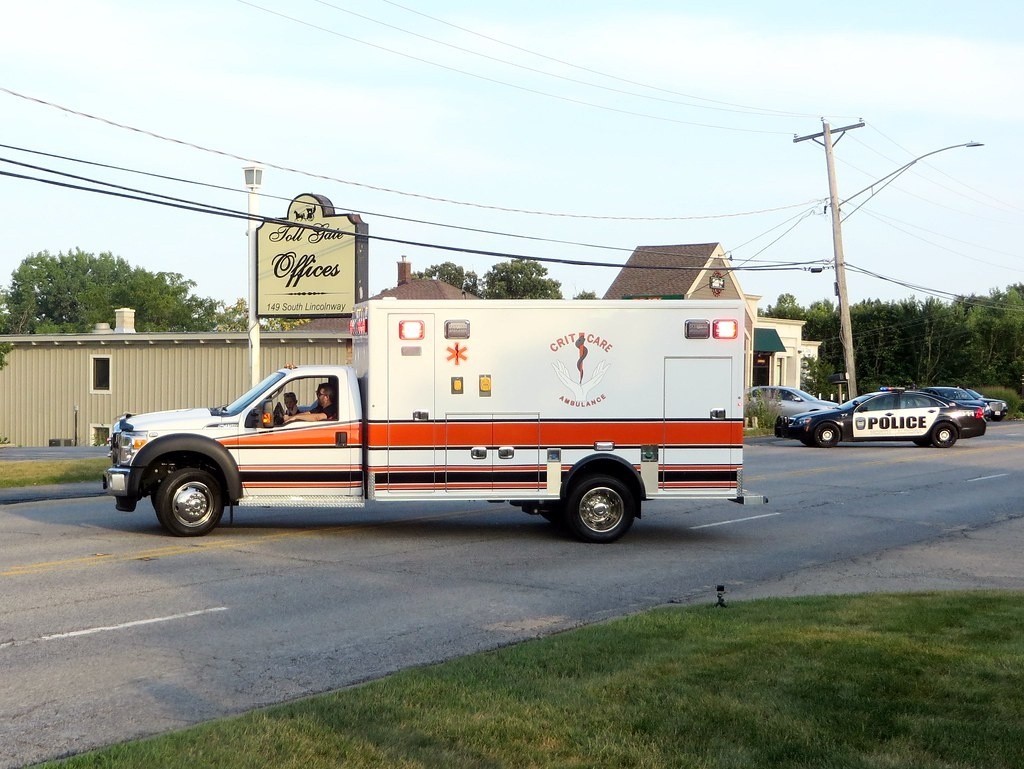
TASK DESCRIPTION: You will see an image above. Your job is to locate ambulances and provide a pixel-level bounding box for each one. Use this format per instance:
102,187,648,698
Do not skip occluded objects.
100,293,770,544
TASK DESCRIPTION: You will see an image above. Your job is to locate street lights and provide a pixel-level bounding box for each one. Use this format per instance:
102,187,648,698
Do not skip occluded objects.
243,162,265,392
829,142,985,396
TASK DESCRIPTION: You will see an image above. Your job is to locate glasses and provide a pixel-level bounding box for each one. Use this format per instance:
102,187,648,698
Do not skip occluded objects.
317,392,326,397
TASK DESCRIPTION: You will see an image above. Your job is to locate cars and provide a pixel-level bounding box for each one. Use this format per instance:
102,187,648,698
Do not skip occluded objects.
774,389,989,450
967,388,1008,422
919,384,992,422
744,383,839,425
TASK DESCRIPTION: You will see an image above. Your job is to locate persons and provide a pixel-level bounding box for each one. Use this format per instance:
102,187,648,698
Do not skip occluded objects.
282,384,336,424
283,392,301,416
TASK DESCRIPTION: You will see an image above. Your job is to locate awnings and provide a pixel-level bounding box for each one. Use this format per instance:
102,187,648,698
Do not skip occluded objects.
754,328,786,352
